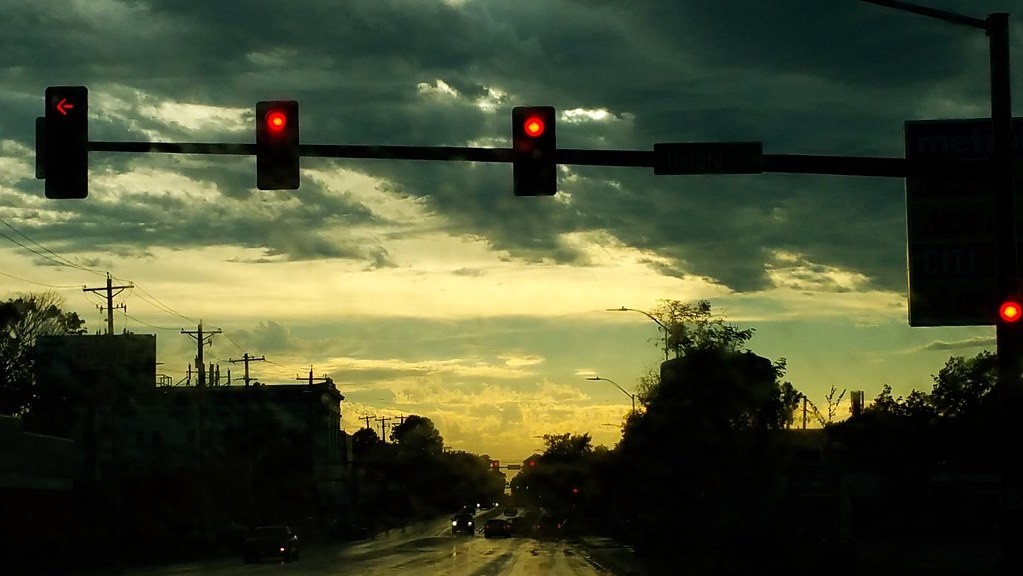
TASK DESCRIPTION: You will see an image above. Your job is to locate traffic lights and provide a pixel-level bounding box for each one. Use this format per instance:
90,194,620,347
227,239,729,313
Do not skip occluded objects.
256,102,300,191
572,487,579,496
492,460,500,472
511,106,558,198
993,295,1022,366
45,86,88,201
527,460,537,472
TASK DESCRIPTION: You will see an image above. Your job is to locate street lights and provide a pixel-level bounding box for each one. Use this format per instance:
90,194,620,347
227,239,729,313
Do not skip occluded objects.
585,376,635,414
606,307,675,361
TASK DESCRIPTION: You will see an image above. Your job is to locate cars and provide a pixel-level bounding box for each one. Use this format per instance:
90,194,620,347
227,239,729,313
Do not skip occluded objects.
240,524,302,565
504,503,564,541
465,501,500,515
450,514,476,535
485,519,511,540
